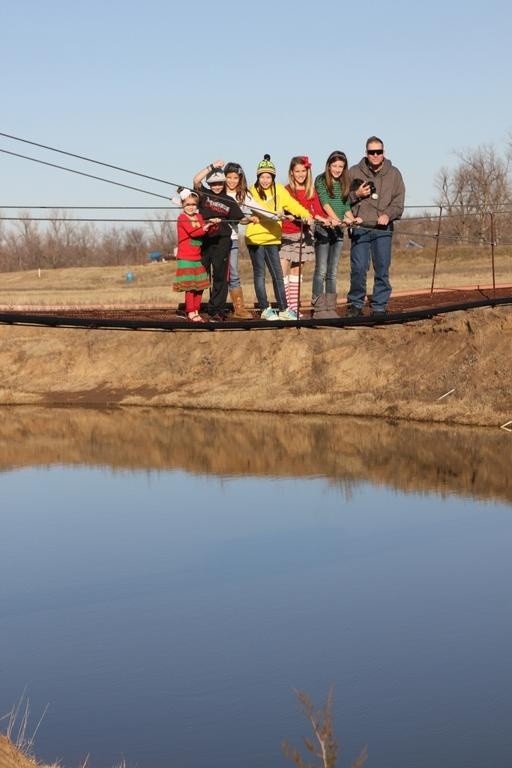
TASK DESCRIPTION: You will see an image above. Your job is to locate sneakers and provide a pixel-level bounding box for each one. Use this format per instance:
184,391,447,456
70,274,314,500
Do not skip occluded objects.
188,313,225,322
279,308,297,320
260,307,279,321
370,311,385,315
347,305,361,317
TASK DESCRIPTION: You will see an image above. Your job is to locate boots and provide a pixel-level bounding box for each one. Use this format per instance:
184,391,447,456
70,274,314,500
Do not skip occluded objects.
230,286,253,320
312,293,339,319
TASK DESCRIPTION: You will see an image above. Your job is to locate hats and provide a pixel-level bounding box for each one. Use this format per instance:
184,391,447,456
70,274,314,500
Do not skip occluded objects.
206,169,226,183
256,154,276,176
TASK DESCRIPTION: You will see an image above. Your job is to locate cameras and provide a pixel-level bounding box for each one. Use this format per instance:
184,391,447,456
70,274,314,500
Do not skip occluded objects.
363,181,376,193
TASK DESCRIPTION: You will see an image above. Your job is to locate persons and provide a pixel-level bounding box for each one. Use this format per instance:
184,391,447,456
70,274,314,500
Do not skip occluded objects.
243,154,315,322
222,162,283,321
347,136,407,319
278,154,342,319
192,159,260,324
171,185,223,324
309,150,364,320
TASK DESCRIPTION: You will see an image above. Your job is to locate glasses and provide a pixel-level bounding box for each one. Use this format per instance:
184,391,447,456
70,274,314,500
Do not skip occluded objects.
367,150,383,155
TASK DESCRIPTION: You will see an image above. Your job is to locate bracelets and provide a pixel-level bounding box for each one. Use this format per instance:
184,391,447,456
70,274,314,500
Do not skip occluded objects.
207,167,211,173
210,164,214,171
